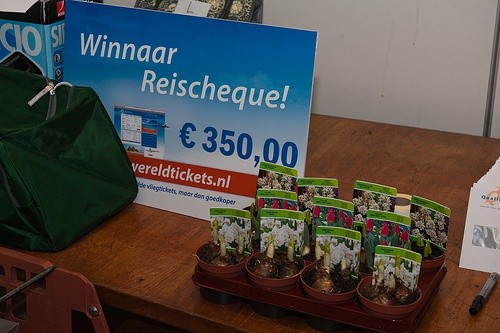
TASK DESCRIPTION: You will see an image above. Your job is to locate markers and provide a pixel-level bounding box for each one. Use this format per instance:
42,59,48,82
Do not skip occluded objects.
468,271,498,314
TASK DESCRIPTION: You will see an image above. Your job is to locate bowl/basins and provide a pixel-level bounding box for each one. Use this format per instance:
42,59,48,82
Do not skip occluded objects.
300,262,363,301
421,247,447,269
245,251,306,287
196,241,254,274
356,276,422,314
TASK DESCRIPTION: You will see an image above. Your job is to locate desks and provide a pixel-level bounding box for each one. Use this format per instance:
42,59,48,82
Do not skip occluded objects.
12,112,500,333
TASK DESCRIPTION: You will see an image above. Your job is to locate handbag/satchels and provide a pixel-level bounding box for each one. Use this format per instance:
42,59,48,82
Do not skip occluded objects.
1,64,139,253
